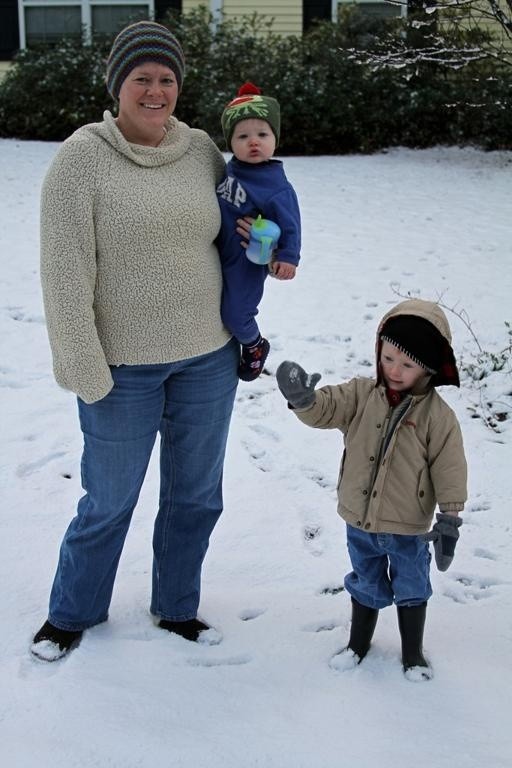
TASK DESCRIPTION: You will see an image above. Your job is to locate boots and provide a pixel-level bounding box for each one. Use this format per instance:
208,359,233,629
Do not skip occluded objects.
397,601,431,681
329,596,379,670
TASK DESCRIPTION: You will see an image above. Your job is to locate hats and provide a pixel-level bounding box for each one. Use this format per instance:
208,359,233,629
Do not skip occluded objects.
106,21,185,100
381,315,443,374
221,83,280,150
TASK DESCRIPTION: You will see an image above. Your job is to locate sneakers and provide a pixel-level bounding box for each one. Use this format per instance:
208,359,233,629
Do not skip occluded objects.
31,619,80,662
158,617,220,645
237,339,270,381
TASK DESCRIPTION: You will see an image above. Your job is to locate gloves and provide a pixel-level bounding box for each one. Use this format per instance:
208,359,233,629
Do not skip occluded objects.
276,361,321,410
422,513,462,572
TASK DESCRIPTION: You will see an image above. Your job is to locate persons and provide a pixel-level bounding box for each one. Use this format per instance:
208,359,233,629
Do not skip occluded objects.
212,81,302,382
26,24,259,661
276,300,469,684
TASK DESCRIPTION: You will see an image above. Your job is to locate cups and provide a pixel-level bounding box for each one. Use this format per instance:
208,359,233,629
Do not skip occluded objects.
243,213,283,267
